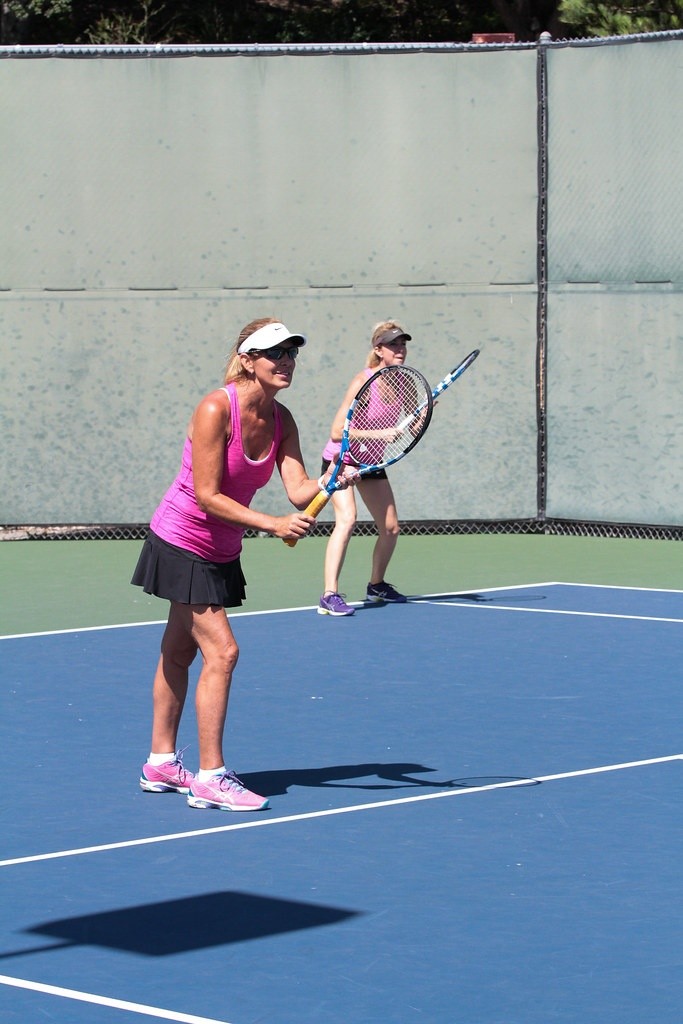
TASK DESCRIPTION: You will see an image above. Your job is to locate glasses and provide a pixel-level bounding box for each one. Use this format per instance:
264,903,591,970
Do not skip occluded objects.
246,346,299,360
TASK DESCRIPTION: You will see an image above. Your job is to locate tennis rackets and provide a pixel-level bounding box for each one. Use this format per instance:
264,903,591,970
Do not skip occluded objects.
282,363,433,548
396,349,480,431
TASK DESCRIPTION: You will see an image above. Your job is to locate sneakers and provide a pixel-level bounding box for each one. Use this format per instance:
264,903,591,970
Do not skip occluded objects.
139,744,196,795
186,769,270,812
367,579,407,603
317,590,355,616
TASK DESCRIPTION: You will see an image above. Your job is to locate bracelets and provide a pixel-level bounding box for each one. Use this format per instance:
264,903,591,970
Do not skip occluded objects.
318,475,324,491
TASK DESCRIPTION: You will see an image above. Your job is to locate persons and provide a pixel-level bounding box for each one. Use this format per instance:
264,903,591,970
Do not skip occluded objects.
131,318,361,811
317,318,438,616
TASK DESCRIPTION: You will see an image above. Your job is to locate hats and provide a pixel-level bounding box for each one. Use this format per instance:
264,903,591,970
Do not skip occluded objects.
238,323,306,353
373,328,412,346
